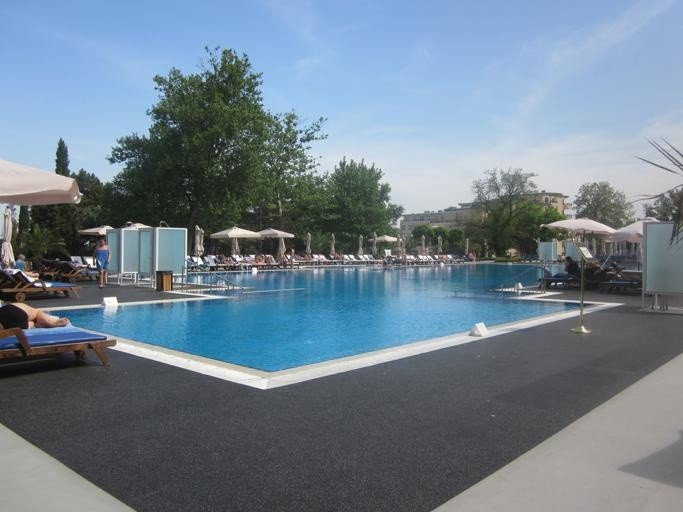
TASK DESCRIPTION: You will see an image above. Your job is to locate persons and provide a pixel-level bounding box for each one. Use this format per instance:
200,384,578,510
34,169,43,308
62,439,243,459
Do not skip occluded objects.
25,257,31,271
16,254,25,270
76,224,113,237
0,303,68,332
93,238,111,287
187,248,295,269
565,257,580,276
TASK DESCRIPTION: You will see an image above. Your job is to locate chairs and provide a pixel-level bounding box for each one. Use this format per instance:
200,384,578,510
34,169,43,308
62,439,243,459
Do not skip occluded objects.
0,255,116,367
538,255,642,294
191,254,465,271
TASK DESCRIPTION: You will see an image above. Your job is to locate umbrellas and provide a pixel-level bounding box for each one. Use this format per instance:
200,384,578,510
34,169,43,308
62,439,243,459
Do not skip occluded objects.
305,232,443,257
540,215,616,243
210,226,261,255
0,159,84,205
616,217,656,271
193,225,204,257
259,228,294,253
1,206,15,268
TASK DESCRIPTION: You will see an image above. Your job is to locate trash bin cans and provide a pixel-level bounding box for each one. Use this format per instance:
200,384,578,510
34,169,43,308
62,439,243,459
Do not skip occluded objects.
156,271,173,291
